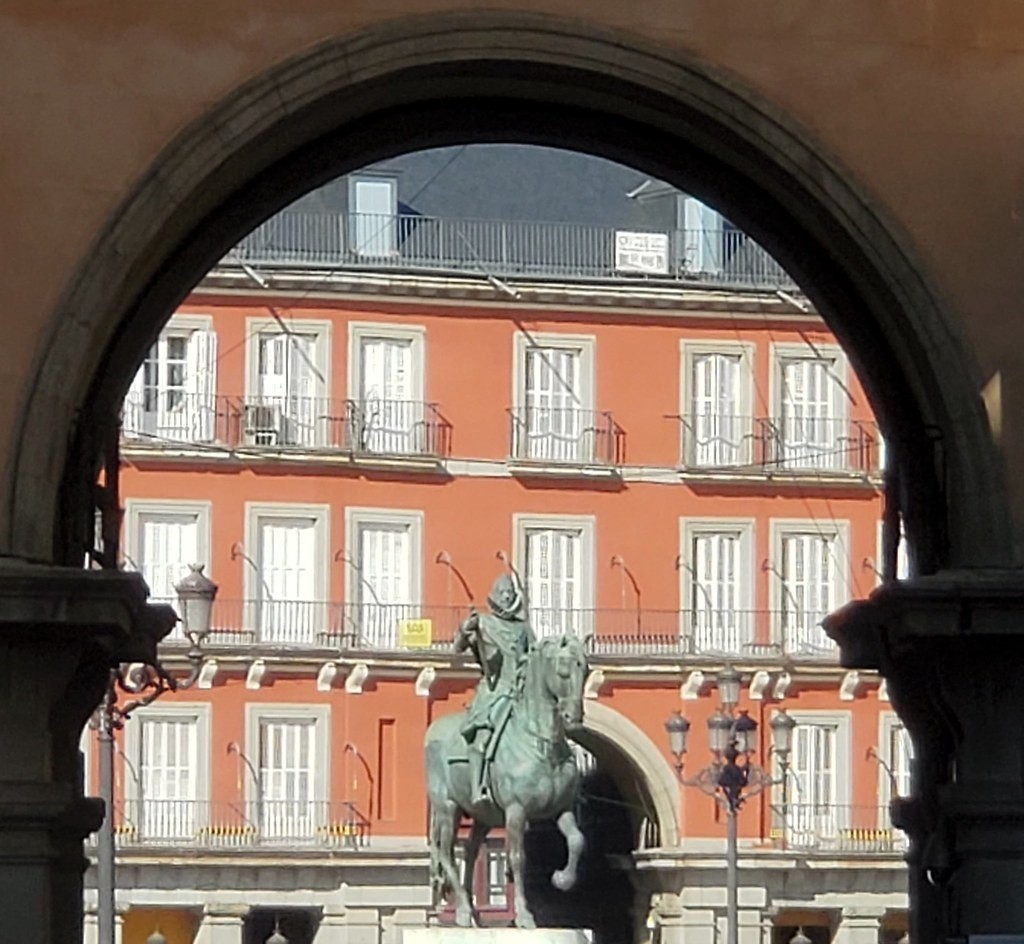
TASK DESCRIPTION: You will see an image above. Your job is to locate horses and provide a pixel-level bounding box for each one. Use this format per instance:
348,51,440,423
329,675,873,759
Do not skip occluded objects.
422,633,595,927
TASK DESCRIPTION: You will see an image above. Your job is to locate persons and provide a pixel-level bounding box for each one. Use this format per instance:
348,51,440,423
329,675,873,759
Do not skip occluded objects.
453,574,539,808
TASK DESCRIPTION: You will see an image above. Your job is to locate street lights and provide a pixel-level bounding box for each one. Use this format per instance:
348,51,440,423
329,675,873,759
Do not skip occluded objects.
89,562,219,944
665,700,796,944
